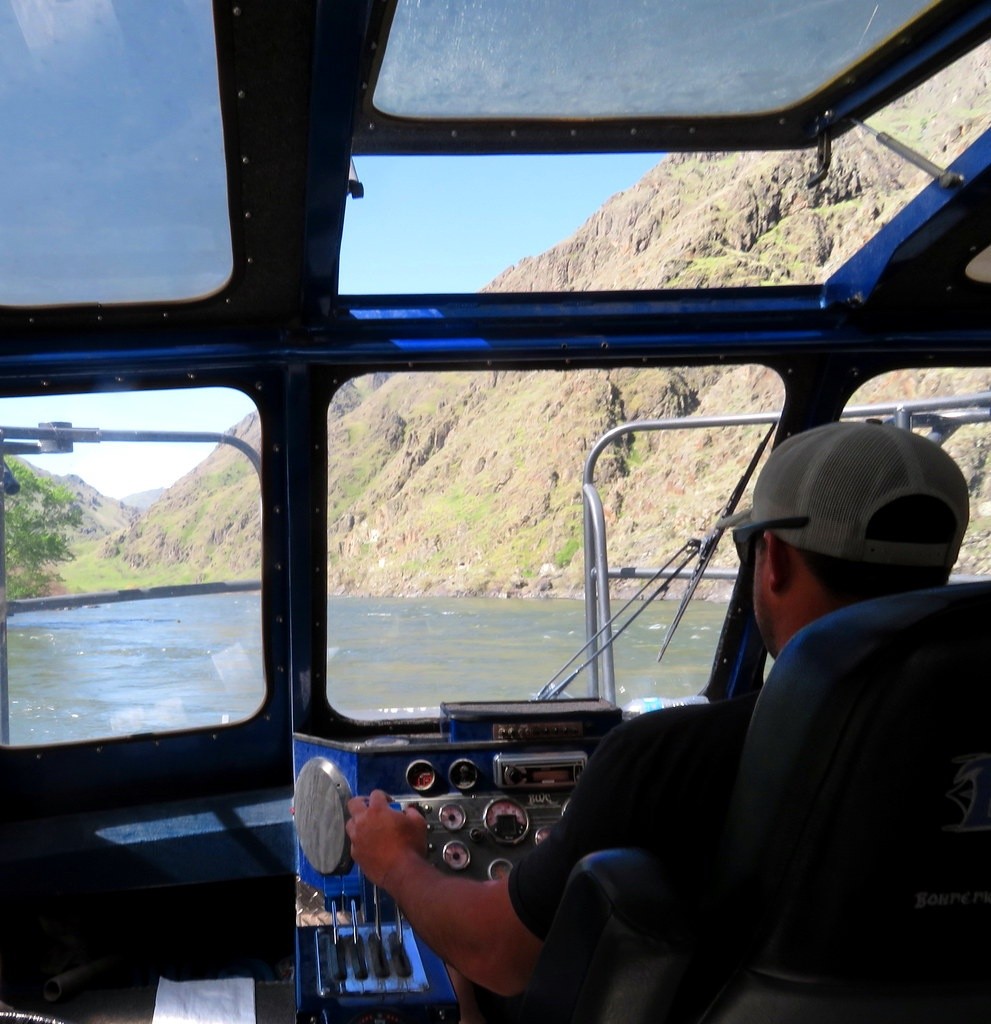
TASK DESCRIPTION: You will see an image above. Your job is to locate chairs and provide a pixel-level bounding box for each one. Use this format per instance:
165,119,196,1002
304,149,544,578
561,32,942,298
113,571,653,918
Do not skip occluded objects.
658,580,990,1023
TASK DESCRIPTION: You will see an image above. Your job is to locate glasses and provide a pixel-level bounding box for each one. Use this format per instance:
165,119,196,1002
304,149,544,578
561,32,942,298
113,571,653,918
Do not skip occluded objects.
731,513,808,564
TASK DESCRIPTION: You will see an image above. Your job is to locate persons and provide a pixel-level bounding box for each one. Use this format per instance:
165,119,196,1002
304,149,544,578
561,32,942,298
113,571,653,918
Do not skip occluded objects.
346,421,971,1024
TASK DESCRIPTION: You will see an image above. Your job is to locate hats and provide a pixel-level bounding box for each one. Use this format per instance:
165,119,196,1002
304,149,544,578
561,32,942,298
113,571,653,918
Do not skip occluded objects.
716,419,970,560
